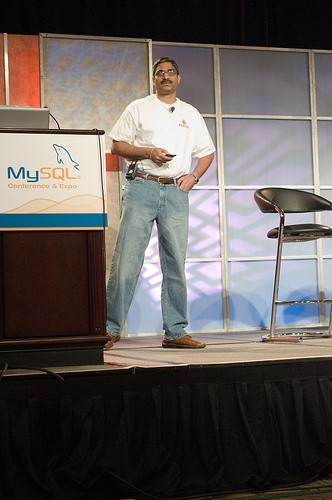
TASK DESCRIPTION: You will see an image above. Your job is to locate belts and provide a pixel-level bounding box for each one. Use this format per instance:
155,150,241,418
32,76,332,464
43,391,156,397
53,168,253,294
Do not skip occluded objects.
136,172,178,184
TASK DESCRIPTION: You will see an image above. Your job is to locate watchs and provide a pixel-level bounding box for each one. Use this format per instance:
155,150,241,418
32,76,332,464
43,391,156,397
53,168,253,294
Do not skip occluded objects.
188,172,199,184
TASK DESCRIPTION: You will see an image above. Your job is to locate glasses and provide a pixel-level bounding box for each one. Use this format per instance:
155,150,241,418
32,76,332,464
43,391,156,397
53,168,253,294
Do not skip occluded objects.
154,69,177,77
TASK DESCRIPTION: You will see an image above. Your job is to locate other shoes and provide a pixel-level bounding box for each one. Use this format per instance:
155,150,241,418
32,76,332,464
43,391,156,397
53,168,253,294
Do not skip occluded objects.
103,332,120,350
162,335,206,349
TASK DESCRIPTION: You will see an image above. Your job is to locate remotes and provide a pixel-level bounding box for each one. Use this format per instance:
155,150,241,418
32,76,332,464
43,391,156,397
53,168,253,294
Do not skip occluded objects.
166,154,176,157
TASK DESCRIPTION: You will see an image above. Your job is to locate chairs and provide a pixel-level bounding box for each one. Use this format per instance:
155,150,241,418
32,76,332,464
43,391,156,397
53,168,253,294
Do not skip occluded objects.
254,186,332,343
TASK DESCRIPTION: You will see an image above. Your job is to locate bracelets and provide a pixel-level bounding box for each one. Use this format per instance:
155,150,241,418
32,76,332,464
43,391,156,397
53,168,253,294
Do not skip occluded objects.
148,148,156,158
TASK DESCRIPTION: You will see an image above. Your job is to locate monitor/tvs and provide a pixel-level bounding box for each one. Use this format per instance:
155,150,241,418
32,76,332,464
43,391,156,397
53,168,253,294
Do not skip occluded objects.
0,106,49,130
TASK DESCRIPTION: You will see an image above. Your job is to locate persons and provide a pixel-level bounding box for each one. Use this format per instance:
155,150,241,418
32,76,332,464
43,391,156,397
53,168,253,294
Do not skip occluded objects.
103,56,216,349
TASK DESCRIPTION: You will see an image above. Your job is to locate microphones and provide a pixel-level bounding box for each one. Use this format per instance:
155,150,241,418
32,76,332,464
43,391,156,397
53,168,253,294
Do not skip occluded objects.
171,107,175,112
